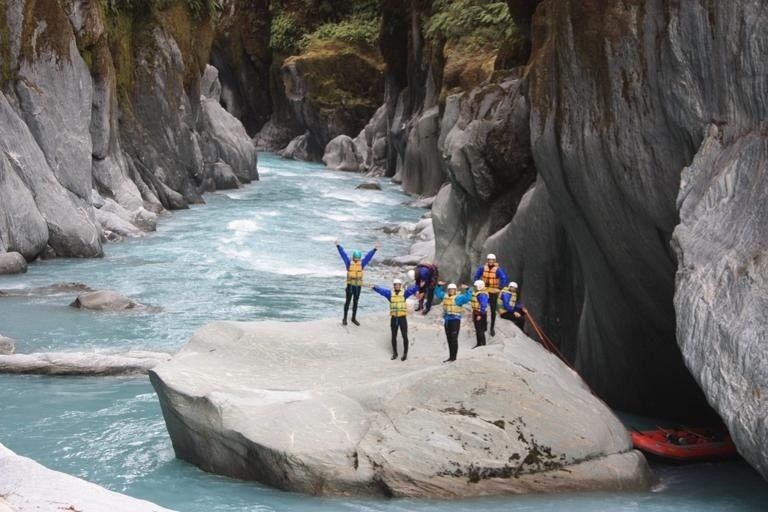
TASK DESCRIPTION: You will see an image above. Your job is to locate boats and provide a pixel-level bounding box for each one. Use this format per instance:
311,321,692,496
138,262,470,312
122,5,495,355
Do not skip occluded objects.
629,422,735,462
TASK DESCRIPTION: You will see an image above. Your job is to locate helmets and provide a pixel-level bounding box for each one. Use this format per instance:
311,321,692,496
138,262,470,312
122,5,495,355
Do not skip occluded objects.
447,283,457,290
487,254,496,263
393,279,401,284
474,280,485,291
353,250,361,259
509,282,518,289
420,268,430,280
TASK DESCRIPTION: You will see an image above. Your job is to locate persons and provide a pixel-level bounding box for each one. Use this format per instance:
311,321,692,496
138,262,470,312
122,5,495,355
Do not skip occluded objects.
497,282,530,336
473,254,507,337
414,264,439,315
369,279,426,361
472,280,489,349
435,281,472,363
335,239,380,325
